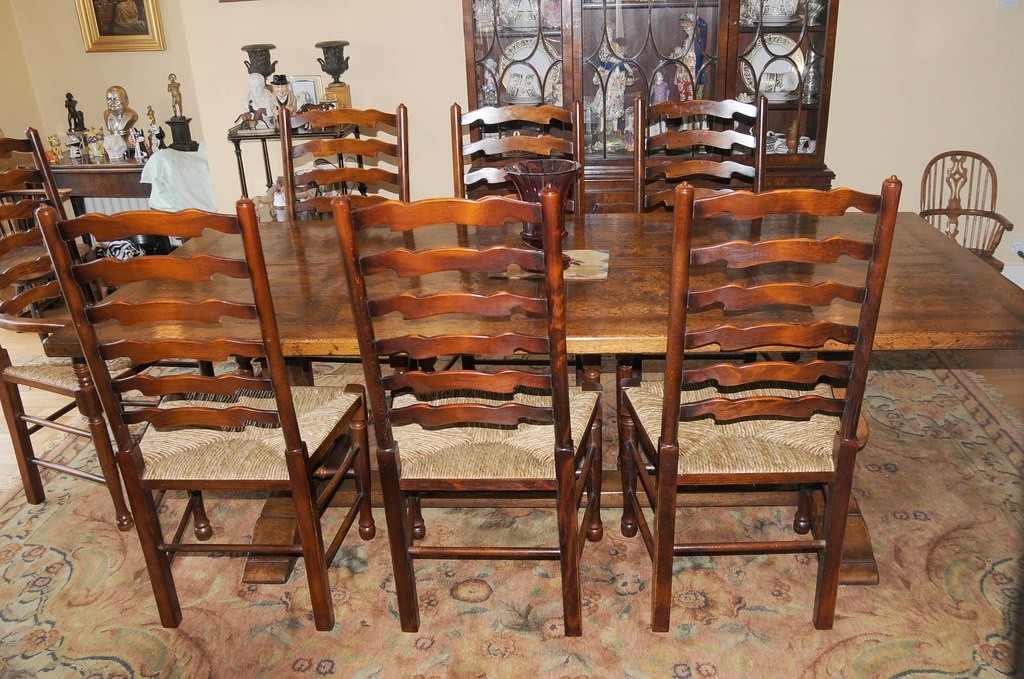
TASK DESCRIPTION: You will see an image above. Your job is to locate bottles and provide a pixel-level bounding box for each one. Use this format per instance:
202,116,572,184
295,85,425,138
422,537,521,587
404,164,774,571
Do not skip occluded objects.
802,45,821,106
801,0,827,27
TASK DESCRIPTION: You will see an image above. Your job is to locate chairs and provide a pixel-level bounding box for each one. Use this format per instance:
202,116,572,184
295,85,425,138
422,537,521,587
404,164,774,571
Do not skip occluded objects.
327,183,604,638
634,95,769,381
450,98,585,387
918,150,1014,274
0,102,409,632
617,174,903,629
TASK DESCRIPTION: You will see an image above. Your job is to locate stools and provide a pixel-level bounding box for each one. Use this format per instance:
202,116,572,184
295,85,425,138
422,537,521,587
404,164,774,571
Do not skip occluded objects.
0,242,108,342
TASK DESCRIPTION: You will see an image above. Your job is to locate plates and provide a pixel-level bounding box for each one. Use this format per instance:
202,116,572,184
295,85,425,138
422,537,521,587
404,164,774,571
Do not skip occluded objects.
740,33,805,95
498,37,561,101
753,20,800,26
494,0,547,25
767,99,795,103
503,98,550,106
503,24,546,31
743,0,799,21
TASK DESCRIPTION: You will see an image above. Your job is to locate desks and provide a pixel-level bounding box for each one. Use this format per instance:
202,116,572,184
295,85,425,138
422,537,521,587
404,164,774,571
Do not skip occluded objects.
43,211,1024,587
18,150,174,253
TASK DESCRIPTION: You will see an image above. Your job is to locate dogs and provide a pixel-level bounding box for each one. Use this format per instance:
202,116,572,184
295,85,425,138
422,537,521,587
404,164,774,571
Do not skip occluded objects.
130,128,149,159
149,126,166,153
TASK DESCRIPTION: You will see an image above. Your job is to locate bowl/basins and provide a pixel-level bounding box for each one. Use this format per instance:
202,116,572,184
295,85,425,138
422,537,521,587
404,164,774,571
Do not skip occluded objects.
758,15,794,21
759,90,793,99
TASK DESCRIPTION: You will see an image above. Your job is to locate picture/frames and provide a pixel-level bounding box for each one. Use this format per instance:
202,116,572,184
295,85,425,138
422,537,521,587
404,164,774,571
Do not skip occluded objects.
288,75,322,111
75,0,168,53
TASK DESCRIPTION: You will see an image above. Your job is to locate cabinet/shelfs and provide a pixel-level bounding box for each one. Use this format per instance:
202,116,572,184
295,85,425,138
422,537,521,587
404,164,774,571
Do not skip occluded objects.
462,0,840,213
227,120,368,223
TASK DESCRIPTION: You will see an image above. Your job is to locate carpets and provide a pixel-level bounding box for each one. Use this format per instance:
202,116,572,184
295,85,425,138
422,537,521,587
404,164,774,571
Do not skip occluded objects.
1,345,1024,678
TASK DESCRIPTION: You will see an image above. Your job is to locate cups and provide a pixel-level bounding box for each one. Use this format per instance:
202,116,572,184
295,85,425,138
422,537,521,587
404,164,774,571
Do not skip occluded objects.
766,130,811,154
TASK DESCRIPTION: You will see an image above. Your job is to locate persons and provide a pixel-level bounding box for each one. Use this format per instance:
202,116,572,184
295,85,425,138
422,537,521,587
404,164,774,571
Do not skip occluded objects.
594,13,708,135
104,86,138,131
238,72,274,128
65,93,78,130
249,100,255,120
164,73,182,118
147,106,155,126
269,75,297,116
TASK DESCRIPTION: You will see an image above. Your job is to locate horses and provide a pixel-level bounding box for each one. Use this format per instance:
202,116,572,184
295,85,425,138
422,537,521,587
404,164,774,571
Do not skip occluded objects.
234,107,269,129
251,184,282,218
276,177,318,216
299,102,334,132
